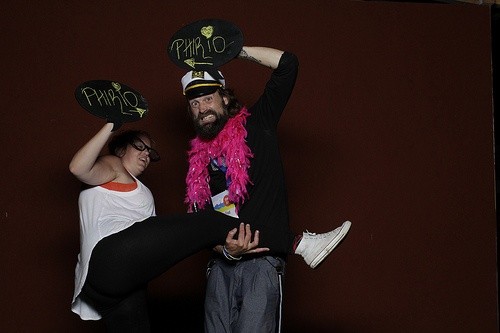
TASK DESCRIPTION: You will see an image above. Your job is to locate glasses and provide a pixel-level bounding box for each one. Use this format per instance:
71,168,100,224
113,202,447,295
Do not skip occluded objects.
126,135,160,162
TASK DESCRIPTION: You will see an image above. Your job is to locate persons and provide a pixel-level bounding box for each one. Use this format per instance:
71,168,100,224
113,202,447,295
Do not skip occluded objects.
68,77,354,327
165,22,294,333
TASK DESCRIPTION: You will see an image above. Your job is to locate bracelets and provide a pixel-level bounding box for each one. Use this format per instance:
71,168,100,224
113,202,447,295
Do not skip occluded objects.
219,245,248,266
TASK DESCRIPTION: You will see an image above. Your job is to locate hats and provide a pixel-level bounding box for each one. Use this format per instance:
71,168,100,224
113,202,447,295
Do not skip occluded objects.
181,68,225,98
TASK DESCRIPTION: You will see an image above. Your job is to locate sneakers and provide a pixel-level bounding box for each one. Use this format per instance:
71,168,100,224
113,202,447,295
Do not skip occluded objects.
294,221,352,269
